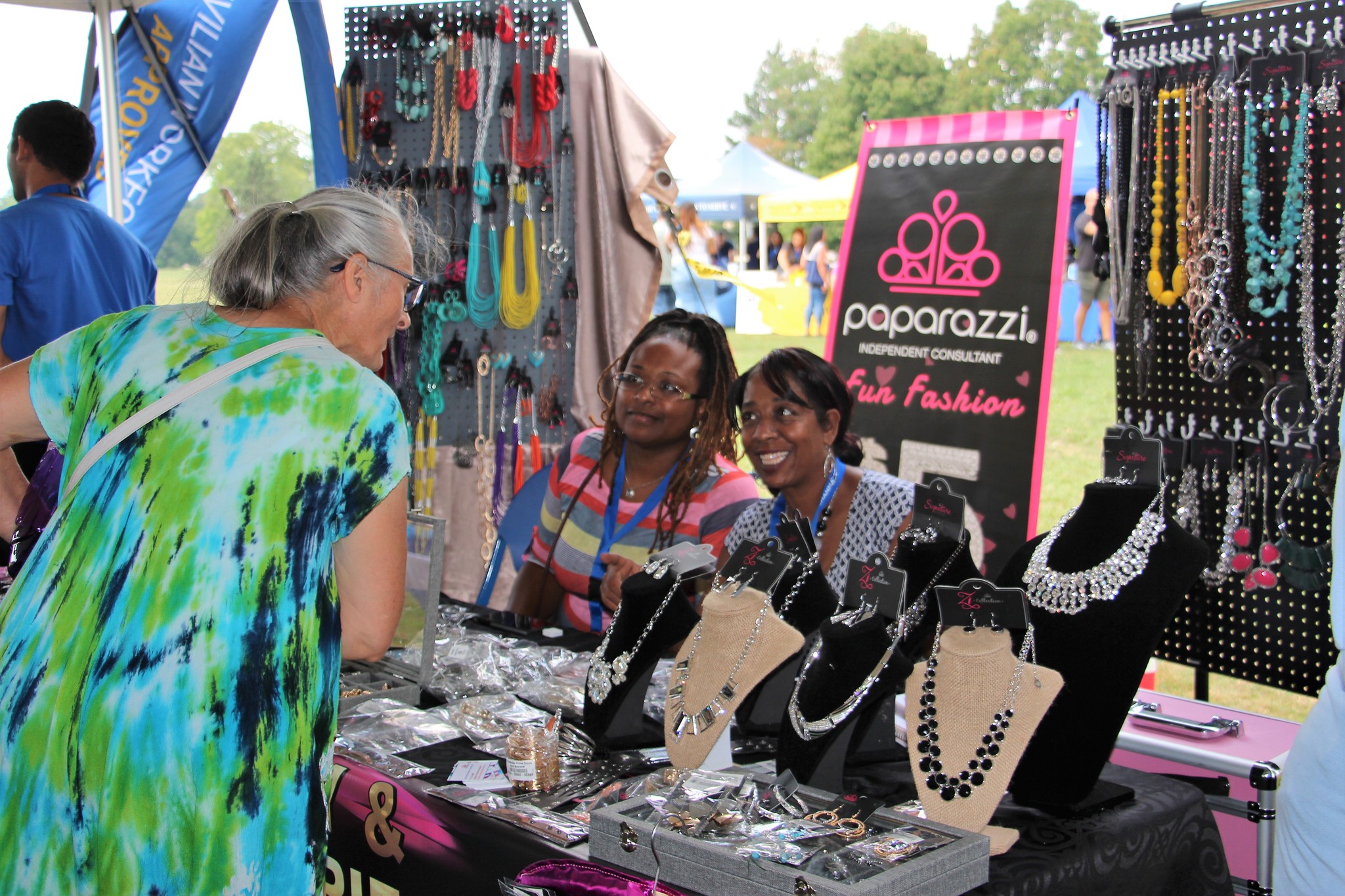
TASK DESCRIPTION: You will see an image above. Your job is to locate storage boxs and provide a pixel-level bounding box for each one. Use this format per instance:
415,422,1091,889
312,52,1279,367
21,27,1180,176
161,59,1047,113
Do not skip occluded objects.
588,766,990,896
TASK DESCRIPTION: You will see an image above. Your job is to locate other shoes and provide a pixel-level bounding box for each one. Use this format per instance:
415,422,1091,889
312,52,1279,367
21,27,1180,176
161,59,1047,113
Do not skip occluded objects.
1073,339,1116,352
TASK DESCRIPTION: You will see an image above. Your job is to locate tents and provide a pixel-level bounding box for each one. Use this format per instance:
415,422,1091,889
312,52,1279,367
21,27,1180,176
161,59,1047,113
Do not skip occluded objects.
757,157,859,273
1054,89,1114,196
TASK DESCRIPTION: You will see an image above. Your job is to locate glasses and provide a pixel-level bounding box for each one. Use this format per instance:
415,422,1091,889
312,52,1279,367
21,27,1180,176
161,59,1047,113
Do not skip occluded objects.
613,372,701,402
331,257,428,313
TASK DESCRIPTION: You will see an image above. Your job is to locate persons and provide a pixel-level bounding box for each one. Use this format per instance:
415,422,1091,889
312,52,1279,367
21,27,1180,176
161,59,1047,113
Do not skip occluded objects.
599,347,915,614
1072,187,1117,355
655,200,721,324
993,482,1210,807
710,221,807,276
0,187,423,895
584,557,843,774
877,523,988,662
774,602,940,795
505,308,760,640
798,221,834,338
0,99,157,568
905,623,1065,832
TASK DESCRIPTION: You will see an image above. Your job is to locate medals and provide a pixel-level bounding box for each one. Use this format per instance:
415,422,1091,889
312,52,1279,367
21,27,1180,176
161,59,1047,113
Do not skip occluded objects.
640,140,819,273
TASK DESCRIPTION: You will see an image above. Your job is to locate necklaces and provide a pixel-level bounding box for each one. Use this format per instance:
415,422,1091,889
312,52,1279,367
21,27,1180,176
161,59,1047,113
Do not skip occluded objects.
1091,48,1345,592
918,622,1036,802
885,535,965,645
29,183,86,199
815,485,838,546
1021,485,1167,615
787,606,900,741
624,470,665,498
586,559,682,705
668,594,772,742
344,1,570,571
776,550,819,621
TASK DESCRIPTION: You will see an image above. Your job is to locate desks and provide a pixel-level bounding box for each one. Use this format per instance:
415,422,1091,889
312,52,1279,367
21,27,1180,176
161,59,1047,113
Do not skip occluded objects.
319,593,1235,896
